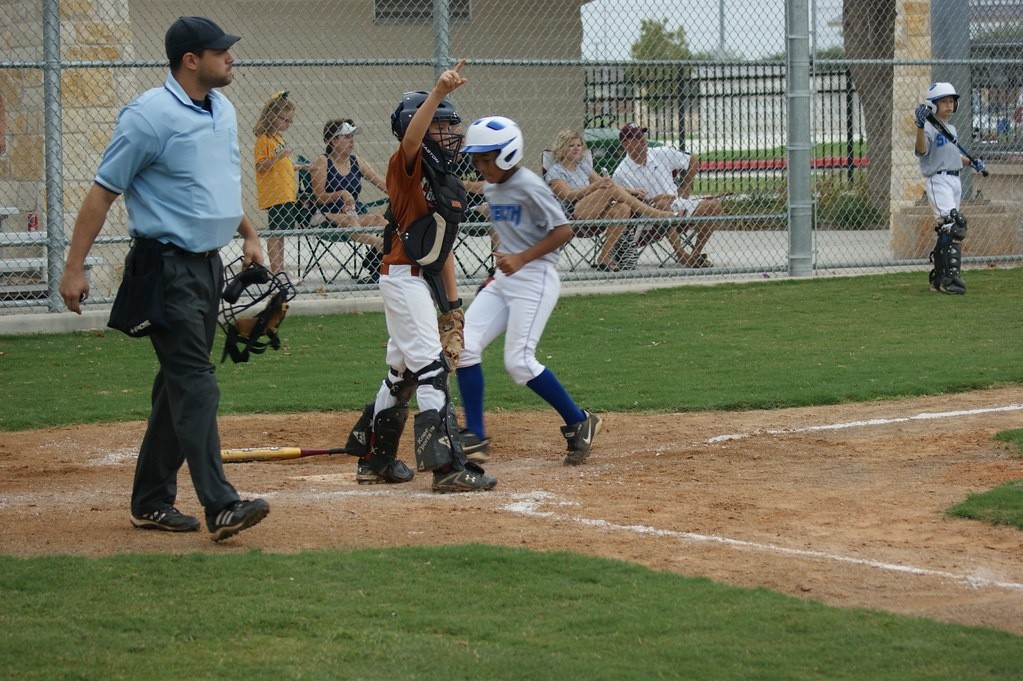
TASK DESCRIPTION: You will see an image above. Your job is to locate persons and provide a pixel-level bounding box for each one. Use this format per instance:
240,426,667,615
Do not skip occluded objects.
612,124,720,268
357,58,498,494
545,130,688,272
311,118,390,280
254,91,315,285
451,123,499,253
914,82,985,295
460,115,604,463
59,15,270,540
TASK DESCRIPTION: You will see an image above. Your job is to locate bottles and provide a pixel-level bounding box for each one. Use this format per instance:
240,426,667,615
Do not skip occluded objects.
345,205,360,222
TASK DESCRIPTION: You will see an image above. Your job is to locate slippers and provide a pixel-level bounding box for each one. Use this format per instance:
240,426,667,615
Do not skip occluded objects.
691,254,714,268
590,262,619,272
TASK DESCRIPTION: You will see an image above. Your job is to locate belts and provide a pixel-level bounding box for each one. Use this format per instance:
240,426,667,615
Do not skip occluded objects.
937,169,960,176
379,263,420,276
135,237,219,260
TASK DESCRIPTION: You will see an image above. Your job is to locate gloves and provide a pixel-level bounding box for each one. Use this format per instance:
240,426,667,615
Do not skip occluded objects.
914,105,931,128
968,158,985,173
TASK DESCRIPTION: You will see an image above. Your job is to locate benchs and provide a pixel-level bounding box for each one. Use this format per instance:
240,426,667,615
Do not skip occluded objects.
0,208,105,293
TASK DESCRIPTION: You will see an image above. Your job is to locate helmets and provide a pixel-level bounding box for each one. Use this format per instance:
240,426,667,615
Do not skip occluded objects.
391,90,464,172
458,116,524,170
925,82,960,114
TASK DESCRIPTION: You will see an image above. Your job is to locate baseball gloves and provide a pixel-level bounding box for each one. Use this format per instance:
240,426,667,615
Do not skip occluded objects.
436,307,466,373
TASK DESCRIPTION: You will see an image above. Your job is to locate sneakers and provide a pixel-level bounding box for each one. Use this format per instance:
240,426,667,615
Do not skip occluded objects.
464,435,493,463
560,408,602,465
431,466,497,494
354,458,414,485
205,497,271,543
930,280,965,295
130,505,201,532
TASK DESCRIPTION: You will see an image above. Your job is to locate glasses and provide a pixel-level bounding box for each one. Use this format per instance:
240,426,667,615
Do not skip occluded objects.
276,114,293,126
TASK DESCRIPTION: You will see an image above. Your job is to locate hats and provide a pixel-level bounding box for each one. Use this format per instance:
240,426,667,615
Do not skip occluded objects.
165,16,242,59
325,121,357,149
619,122,648,141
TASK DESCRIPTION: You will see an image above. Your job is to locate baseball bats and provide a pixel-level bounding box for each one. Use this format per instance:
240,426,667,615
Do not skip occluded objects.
921,103,990,178
220,445,345,462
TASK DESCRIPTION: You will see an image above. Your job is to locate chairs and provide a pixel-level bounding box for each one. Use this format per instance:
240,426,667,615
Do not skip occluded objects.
294,154,733,286
